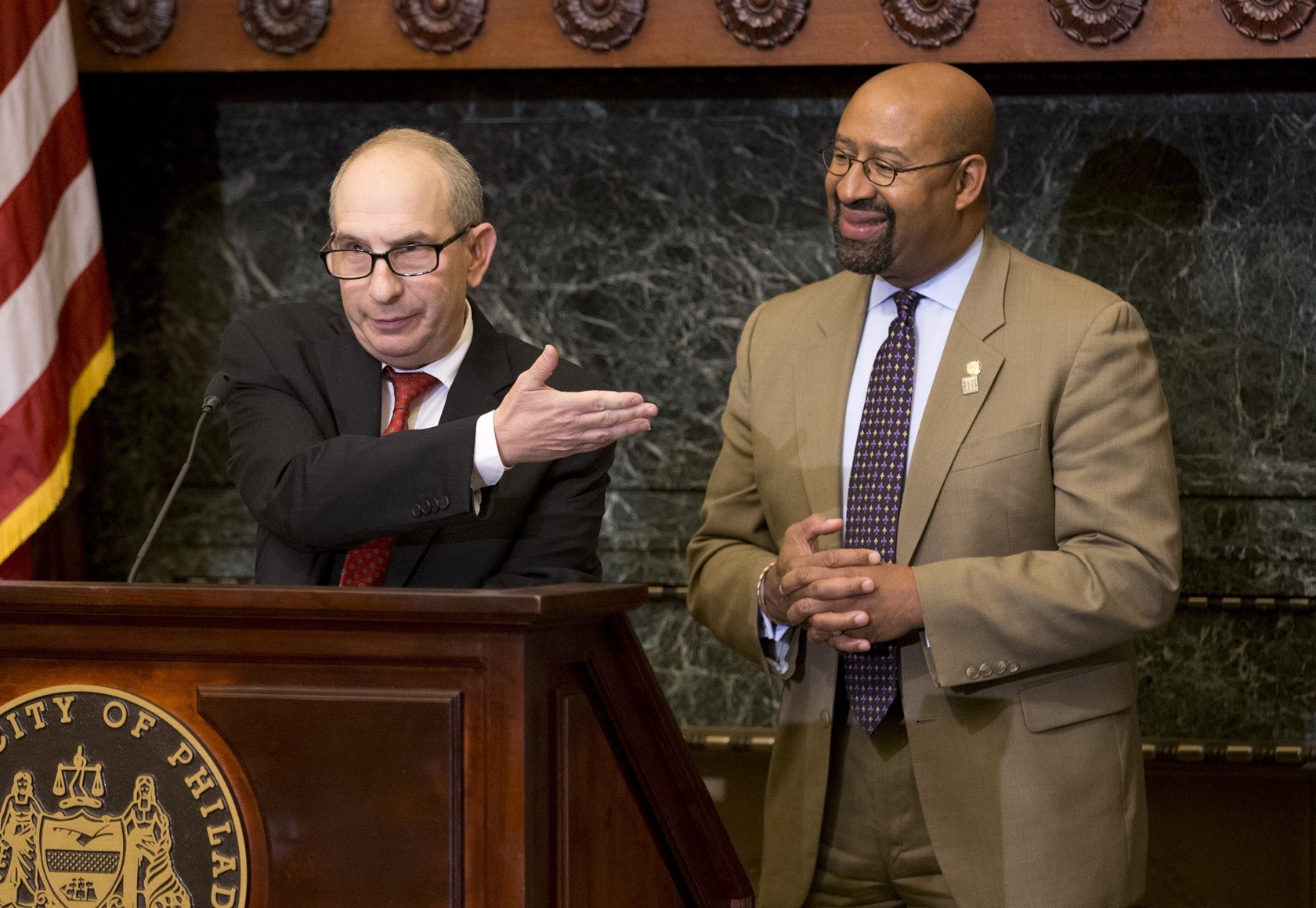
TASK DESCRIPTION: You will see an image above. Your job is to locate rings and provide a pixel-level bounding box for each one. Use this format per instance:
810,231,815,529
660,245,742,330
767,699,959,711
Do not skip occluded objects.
833,630,842,636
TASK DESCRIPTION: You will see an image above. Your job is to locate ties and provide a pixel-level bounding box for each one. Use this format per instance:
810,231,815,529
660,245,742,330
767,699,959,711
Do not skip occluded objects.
843,287,928,737
341,366,440,587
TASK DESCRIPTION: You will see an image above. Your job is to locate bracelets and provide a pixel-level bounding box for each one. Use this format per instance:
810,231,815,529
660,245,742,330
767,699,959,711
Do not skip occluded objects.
756,562,790,626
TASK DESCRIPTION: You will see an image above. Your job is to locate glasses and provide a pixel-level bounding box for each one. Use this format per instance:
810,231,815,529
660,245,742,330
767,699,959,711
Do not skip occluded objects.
822,143,977,187
318,228,475,281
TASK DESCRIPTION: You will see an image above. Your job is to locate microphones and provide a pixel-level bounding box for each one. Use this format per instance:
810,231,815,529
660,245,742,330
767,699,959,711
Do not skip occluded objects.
200,370,234,419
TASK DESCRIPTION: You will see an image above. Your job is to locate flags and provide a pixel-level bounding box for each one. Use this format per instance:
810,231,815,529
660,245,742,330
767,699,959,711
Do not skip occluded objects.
0,0,115,571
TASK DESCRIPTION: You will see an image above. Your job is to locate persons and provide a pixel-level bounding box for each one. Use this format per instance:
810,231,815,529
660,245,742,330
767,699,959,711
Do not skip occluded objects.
684,61,1182,908
214,128,658,589
